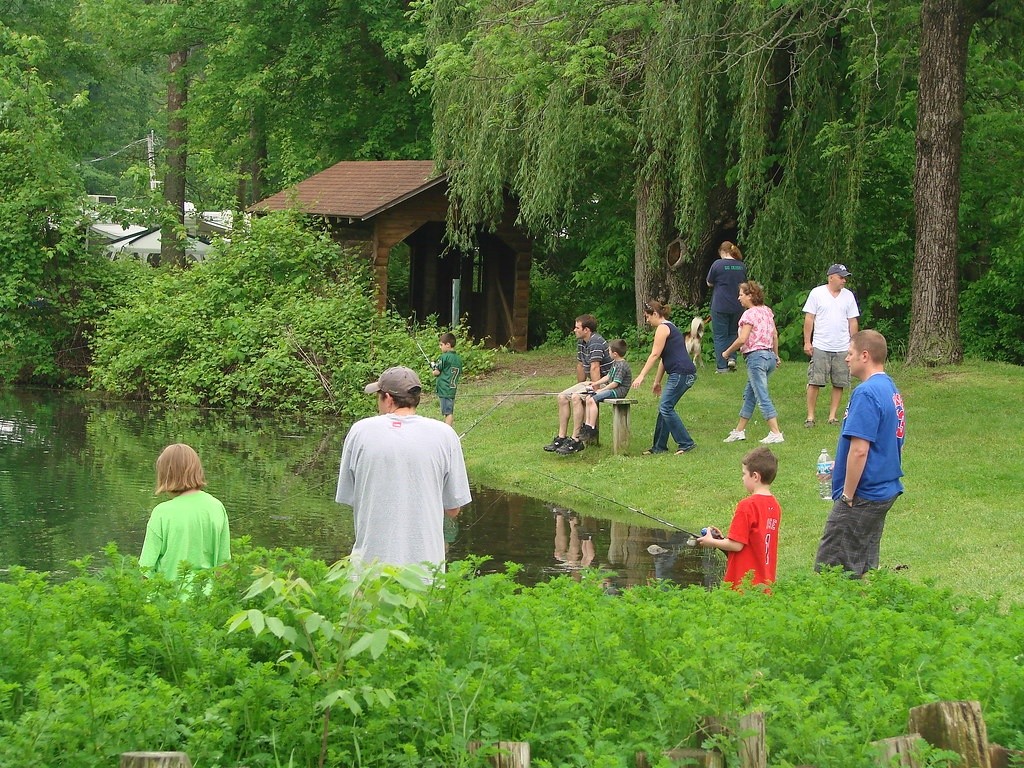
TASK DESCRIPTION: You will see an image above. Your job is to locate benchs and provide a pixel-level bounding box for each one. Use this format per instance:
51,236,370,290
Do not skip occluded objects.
582,398,638,454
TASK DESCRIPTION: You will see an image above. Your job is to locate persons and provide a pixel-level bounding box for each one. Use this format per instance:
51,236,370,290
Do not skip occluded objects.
697,447,782,595
544,315,615,454
633,300,697,455
722,281,786,443
803,264,860,427
334,366,472,594
139,444,233,602
705,241,748,373
812,329,904,580
430,333,463,425
576,339,632,441
551,508,690,592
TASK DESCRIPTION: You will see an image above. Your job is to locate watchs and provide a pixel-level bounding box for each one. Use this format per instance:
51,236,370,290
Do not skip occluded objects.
839,494,853,503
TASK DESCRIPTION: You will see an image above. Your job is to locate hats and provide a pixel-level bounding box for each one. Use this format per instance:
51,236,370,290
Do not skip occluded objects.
364,365,421,395
827,264,852,277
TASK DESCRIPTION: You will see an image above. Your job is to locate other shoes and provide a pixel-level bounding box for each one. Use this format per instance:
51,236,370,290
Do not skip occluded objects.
804,418,816,429
727,361,737,372
642,449,654,454
824,418,841,426
674,444,697,455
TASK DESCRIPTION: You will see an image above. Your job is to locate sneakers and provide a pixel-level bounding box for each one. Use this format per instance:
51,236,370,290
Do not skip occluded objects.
576,423,597,441
556,436,585,455
758,431,785,443
722,428,745,442
543,436,569,452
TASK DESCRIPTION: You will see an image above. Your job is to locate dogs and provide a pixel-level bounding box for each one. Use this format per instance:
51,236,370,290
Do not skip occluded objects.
680,317,705,368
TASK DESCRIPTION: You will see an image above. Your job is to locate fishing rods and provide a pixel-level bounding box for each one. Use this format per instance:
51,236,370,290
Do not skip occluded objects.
526,466,728,557
370,277,437,374
457,371,539,439
431,386,597,398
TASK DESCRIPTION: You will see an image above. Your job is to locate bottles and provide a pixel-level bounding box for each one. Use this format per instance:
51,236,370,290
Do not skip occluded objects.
817,449,835,499
